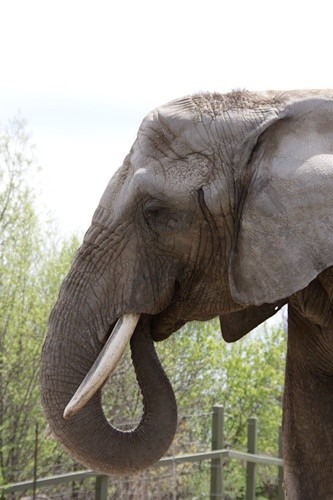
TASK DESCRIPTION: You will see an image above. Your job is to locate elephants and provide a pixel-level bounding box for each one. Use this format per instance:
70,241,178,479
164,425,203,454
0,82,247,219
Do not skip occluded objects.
38,89,331,500
